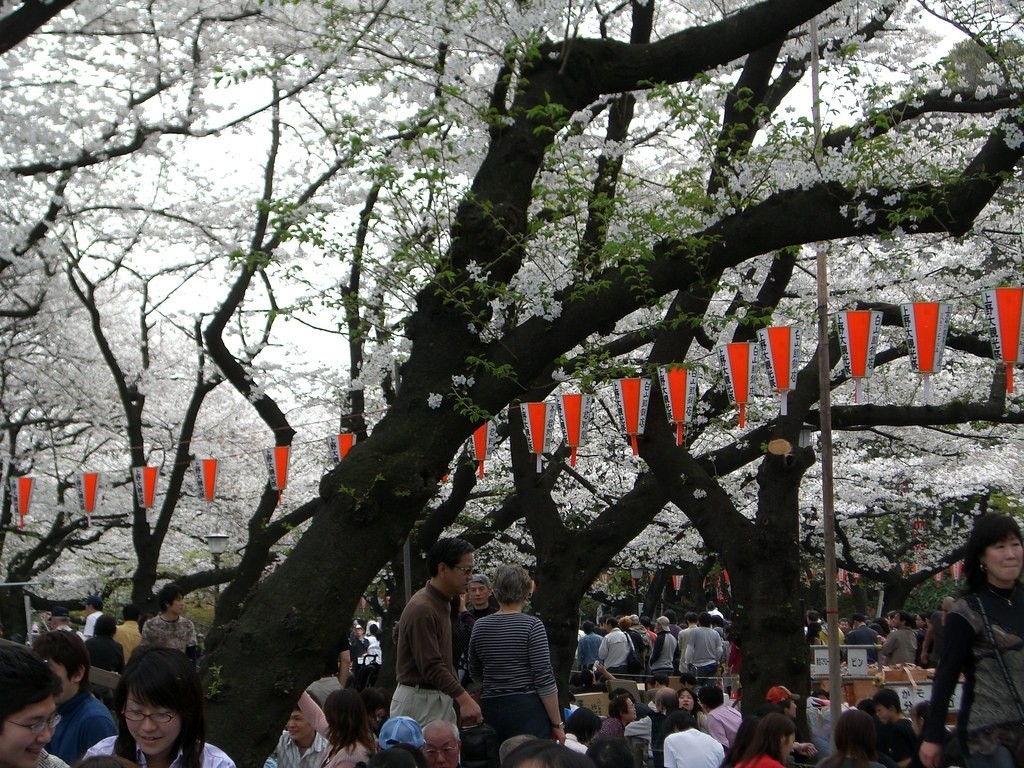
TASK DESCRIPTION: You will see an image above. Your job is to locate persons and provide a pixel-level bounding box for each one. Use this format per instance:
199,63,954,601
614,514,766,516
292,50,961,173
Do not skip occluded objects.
0,513,1024,768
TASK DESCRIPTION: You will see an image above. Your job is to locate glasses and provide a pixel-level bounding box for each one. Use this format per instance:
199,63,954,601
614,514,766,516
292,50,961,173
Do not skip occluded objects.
3,713,62,734
122,702,177,723
453,565,472,574
424,743,457,757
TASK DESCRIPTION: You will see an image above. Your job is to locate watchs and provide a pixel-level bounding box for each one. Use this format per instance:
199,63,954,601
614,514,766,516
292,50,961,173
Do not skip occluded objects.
551,721,564,729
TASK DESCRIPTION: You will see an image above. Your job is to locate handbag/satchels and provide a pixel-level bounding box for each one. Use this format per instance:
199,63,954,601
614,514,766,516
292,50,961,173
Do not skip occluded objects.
628,650,643,672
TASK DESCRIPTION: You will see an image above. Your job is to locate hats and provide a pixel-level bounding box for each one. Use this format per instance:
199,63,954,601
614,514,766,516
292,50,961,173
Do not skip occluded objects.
378,715,426,751
657,616,670,631
766,686,800,705
47,606,69,621
81,596,102,606
629,614,639,624
